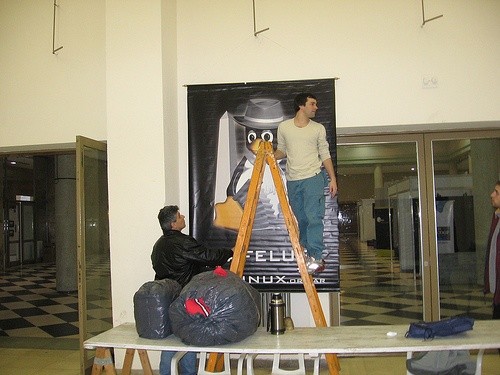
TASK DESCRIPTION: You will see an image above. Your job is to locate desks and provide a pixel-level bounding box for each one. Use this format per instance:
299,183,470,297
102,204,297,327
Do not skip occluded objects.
84,320,500,375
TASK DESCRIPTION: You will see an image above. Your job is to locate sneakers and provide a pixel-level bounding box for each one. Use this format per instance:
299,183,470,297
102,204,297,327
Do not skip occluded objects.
304,252,325,276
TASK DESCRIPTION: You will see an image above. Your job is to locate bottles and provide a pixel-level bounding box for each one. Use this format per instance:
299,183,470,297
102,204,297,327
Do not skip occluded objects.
270,293,285,335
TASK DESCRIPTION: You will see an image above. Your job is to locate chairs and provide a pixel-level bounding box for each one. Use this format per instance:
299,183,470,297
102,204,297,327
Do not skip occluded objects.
171,349,486,375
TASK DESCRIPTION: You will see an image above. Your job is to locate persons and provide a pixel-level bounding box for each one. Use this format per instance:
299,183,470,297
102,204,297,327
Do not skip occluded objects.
269,93,337,274
485,181,500,320
150,205,235,375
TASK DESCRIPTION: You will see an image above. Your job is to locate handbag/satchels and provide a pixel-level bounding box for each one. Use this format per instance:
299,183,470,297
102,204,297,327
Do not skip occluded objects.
408,317,474,338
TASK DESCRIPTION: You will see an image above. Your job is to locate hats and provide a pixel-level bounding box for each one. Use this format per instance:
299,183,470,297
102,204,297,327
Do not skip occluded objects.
230,98,289,129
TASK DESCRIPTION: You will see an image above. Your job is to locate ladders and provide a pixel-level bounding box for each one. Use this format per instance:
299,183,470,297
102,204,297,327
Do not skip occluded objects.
205,139,342,375
